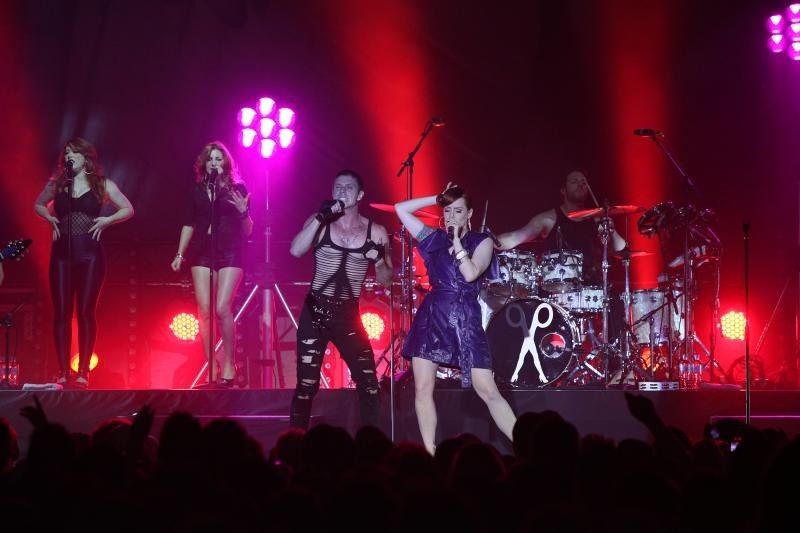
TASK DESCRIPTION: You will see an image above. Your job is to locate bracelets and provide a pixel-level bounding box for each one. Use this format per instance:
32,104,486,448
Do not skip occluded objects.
454,249,469,260
175,252,183,258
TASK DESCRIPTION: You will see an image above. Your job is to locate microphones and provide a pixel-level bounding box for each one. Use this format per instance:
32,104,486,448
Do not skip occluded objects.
432,117,447,127
634,128,663,139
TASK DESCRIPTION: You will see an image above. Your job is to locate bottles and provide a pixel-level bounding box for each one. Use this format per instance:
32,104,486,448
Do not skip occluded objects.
461,368,471,387
348,367,357,388
0,356,19,384
680,354,703,389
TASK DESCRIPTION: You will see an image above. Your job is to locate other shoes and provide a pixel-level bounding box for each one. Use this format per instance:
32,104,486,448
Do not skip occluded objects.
55,380,70,388
72,381,89,390
220,378,234,388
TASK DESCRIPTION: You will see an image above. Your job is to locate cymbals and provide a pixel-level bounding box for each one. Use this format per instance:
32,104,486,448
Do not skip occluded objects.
566,200,645,219
369,202,438,219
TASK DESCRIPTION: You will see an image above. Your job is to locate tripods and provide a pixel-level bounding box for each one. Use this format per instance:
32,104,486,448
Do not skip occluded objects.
560,138,725,390
370,123,414,382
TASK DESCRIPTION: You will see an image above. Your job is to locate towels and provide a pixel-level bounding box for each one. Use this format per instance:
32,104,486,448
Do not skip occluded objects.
23,383,63,390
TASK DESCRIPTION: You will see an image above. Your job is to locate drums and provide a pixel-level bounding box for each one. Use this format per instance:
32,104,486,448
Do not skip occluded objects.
537,251,585,292
624,287,691,347
485,252,539,298
547,286,611,313
486,298,578,391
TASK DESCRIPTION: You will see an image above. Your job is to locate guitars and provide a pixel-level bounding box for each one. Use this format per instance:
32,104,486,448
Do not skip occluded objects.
1,232,32,264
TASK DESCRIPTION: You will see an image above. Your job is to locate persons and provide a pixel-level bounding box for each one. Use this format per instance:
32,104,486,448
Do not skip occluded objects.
288,169,394,434
492,170,626,377
394,181,518,456
0,391,800,533
170,140,255,386
34,136,135,387
